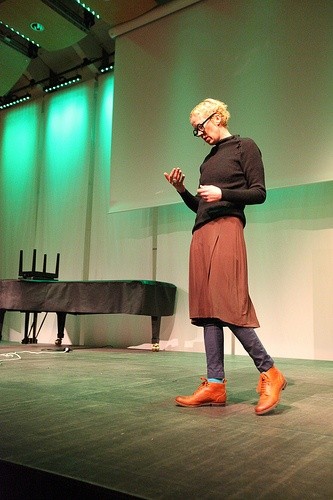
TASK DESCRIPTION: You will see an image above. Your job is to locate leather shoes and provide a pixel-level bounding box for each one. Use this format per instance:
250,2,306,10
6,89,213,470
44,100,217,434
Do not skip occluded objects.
175,376,227,408
254,365,289,417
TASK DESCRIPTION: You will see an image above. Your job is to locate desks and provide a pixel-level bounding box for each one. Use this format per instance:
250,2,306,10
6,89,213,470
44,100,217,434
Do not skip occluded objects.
0,279,177,352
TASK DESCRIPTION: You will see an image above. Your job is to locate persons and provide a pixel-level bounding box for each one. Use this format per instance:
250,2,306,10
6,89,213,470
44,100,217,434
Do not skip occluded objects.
163,98,288,414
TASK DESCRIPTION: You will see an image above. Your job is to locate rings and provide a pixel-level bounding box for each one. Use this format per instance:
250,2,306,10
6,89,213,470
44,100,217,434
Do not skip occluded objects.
173,178,178,184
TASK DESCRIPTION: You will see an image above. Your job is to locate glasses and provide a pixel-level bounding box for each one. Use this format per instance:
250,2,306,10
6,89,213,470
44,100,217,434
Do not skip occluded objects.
192,113,216,137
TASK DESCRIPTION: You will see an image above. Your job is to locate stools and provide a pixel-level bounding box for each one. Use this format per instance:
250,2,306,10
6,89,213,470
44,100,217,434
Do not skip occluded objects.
18,249,60,280
